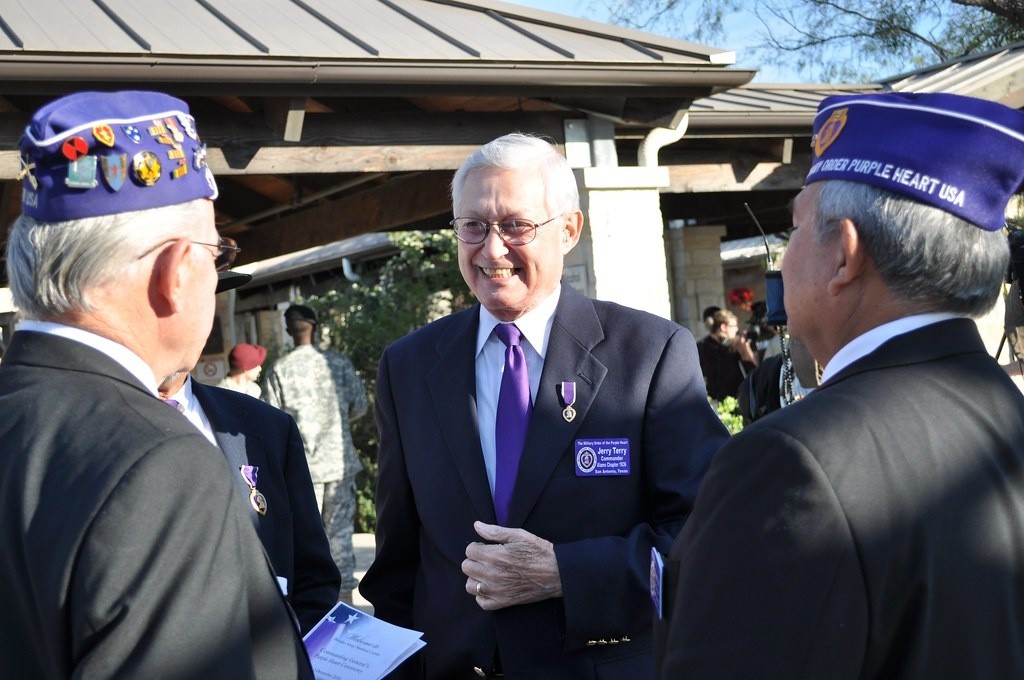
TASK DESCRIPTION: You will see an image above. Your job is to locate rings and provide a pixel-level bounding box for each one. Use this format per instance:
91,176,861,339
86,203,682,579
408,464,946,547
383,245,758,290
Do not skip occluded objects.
477,581,483,596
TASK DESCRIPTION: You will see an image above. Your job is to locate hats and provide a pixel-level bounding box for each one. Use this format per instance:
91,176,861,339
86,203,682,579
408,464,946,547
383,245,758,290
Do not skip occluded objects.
284,305,317,327
227,343,268,376
803,92,1024,231
17,91,220,223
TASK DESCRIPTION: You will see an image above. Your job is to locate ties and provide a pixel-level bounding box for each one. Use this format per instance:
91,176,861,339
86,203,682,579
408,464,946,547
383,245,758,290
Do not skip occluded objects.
493,322,534,529
161,400,181,410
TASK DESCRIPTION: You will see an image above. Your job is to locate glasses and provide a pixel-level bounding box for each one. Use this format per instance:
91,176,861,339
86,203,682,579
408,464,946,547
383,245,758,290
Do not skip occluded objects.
449,212,568,247
138,238,241,273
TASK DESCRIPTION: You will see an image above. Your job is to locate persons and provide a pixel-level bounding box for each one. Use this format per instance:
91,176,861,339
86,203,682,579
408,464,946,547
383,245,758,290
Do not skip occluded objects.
0,91,316,680
264,304,368,606
653,92,1024,680
215,343,267,400
358,133,731,680
158,371,341,639
697,301,767,414
